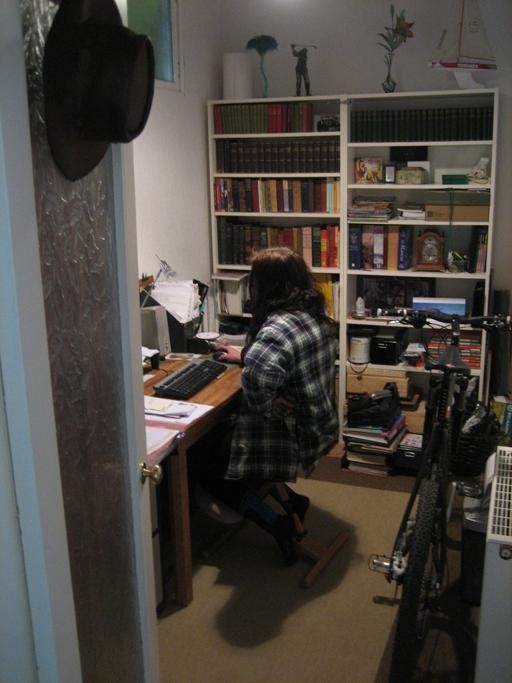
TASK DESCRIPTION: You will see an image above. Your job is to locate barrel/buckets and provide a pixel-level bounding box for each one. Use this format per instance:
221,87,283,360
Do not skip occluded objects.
348,335,371,364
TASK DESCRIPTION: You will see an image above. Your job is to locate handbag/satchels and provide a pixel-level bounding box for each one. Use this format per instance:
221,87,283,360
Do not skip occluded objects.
454,400,501,475
348,380,404,428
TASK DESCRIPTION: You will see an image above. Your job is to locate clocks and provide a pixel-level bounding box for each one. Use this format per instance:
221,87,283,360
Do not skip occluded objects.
413,231,446,272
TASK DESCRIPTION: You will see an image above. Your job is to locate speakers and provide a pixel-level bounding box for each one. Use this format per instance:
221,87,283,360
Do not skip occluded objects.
141,305,172,356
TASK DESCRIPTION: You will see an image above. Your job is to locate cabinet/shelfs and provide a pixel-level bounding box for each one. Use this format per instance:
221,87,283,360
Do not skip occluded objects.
205,97,343,450
343,87,498,434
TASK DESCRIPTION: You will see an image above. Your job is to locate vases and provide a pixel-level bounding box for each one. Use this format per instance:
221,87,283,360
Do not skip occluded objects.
383,64,395,92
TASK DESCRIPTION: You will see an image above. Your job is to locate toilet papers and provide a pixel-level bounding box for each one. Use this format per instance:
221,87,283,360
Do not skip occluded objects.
222,52,254,99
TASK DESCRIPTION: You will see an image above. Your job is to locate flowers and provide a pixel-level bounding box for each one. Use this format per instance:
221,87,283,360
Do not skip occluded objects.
376,3,415,90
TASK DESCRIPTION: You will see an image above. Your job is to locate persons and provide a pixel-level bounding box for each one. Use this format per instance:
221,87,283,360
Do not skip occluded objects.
290,42,311,96
193,243,339,568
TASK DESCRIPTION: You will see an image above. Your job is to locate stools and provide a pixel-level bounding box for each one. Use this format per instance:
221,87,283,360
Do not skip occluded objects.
202,467,351,588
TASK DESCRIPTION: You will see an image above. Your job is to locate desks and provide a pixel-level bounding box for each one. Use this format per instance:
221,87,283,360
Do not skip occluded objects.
145,353,243,607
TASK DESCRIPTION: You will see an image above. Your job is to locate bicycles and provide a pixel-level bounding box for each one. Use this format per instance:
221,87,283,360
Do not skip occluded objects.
368,307,512,683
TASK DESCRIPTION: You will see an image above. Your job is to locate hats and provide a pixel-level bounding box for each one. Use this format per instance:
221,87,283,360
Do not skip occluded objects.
39,0,159,182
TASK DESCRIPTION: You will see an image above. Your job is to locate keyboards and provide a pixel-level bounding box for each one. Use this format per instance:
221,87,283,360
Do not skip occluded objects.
152,358,227,400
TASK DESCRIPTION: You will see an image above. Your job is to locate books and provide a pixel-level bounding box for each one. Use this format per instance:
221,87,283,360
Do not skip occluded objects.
217,216,341,268
342,414,408,477
350,103,493,142
211,102,314,135
144,394,214,463
214,177,340,213
489,391,511,435
427,330,482,368
215,137,341,174
348,224,412,272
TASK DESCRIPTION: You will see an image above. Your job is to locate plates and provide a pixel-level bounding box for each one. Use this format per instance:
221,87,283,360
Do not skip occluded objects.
196,331,219,340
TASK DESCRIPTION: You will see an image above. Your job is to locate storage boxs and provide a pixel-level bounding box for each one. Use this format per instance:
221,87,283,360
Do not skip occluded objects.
461,487,489,607
370,327,406,364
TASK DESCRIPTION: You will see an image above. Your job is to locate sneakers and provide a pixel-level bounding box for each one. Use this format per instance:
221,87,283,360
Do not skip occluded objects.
277,493,310,565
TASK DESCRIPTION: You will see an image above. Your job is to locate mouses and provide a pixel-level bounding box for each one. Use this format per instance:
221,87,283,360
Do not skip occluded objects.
212,352,228,360
